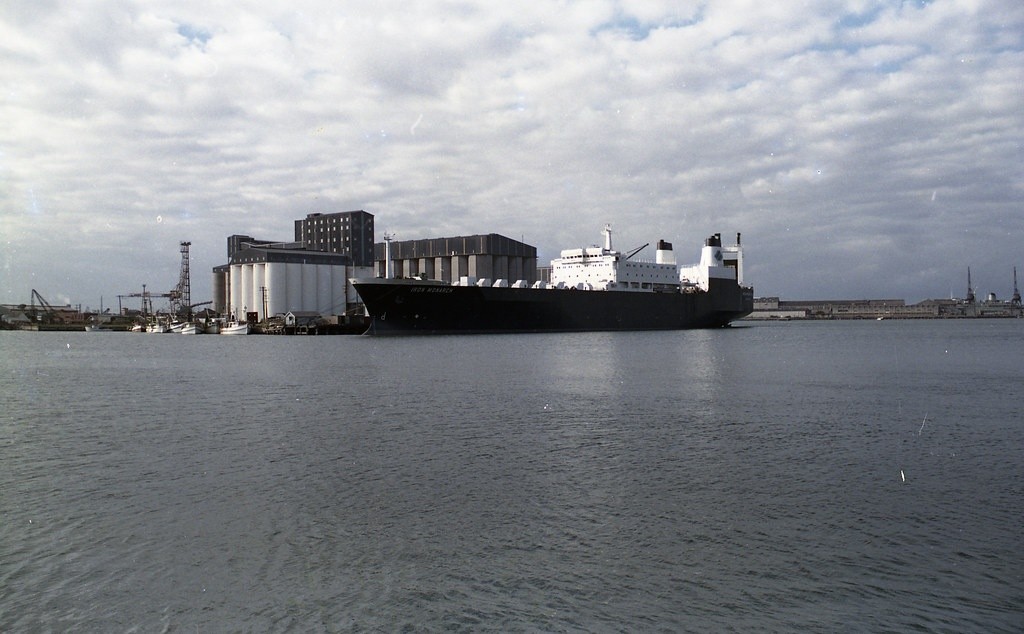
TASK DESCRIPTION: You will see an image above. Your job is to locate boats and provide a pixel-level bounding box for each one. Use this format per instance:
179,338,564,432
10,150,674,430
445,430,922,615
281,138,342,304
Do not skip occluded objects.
132,325,145,332
219,322,247,334
168,319,185,333
181,322,204,334
145,323,167,333
85,326,113,332
205,322,220,334
348,223,754,336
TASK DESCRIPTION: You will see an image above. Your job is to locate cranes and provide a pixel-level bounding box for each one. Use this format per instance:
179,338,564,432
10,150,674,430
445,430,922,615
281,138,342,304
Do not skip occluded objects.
116,284,172,316
